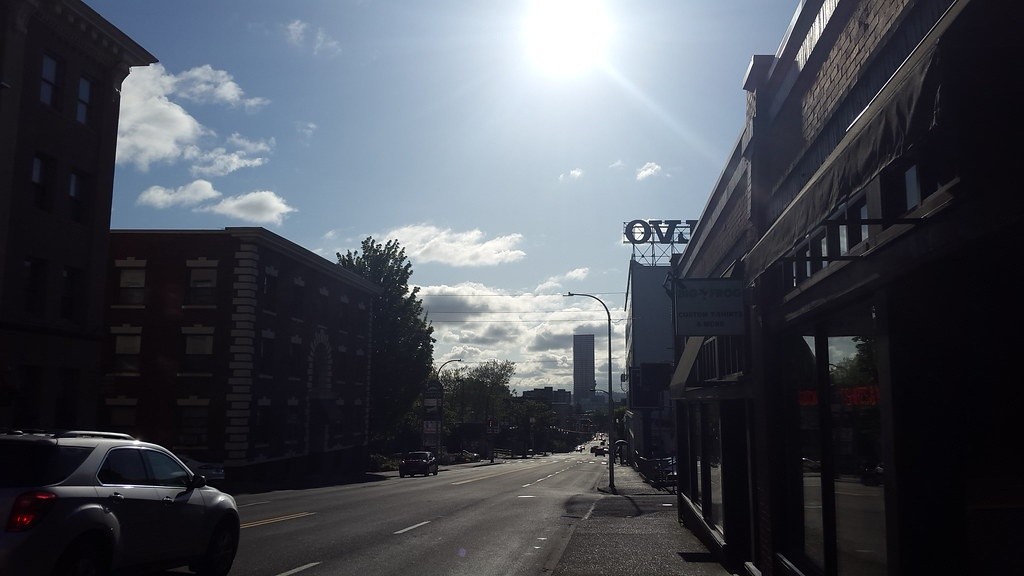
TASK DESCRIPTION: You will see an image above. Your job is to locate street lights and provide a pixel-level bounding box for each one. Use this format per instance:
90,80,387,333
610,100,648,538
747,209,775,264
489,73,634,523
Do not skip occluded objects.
435,360,462,458
562,293,617,490
588,388,616,432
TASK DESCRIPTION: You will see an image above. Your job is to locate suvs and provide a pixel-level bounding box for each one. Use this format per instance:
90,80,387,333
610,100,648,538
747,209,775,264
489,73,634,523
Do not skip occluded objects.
594,447,605,458
0,430,241,576
398,451,440,477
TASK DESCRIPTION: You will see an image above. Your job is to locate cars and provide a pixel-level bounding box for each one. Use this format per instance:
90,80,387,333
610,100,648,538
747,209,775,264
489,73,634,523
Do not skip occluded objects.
576,433,609,453
